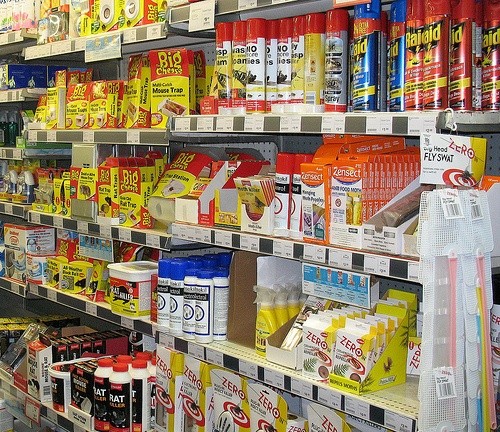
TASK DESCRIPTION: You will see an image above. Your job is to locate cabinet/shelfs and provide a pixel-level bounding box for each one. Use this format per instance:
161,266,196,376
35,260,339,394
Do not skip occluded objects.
0,0,500,432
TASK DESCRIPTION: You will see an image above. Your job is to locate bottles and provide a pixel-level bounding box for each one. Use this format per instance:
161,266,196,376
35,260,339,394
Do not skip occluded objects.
154,249,234,343
209,1,500,114
93,349,158,432
